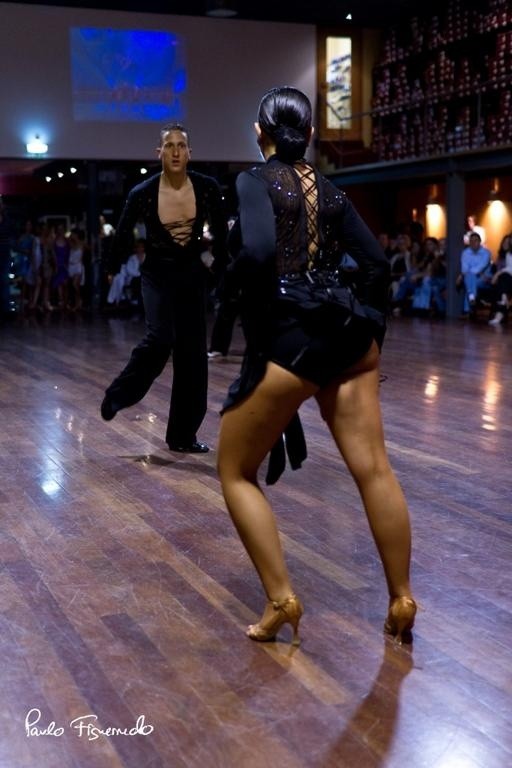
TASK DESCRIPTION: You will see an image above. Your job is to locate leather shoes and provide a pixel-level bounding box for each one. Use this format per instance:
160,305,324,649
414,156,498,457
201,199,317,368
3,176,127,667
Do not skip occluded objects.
101,395,118,420
169,438,209,453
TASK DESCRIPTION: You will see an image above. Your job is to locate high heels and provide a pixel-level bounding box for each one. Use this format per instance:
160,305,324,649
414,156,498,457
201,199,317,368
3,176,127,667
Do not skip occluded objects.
246,597,303,643
383,597,416,645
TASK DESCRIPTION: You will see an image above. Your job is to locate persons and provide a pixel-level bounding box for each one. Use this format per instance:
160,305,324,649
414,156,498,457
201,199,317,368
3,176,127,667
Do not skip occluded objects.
218,89,416,648
16,217,512,360
101,123,229,454
